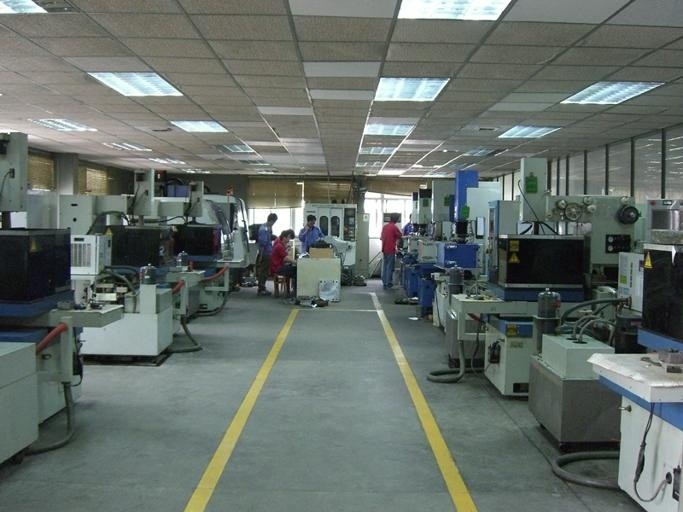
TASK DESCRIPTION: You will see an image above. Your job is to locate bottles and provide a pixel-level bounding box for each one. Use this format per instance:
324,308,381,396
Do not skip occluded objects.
558,213,566,235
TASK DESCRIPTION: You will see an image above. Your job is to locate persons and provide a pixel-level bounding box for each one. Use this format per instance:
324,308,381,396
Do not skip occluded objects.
255,214,278,296
380,214,402,288
274,229,296,266
298,215,325,253
271,231,297,292
403,214,418,235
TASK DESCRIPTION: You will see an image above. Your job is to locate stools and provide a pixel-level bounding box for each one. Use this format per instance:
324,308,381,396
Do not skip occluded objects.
274,274,290,298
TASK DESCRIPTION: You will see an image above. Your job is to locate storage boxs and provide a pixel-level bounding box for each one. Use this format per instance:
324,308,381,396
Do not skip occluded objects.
309,247,335,258
